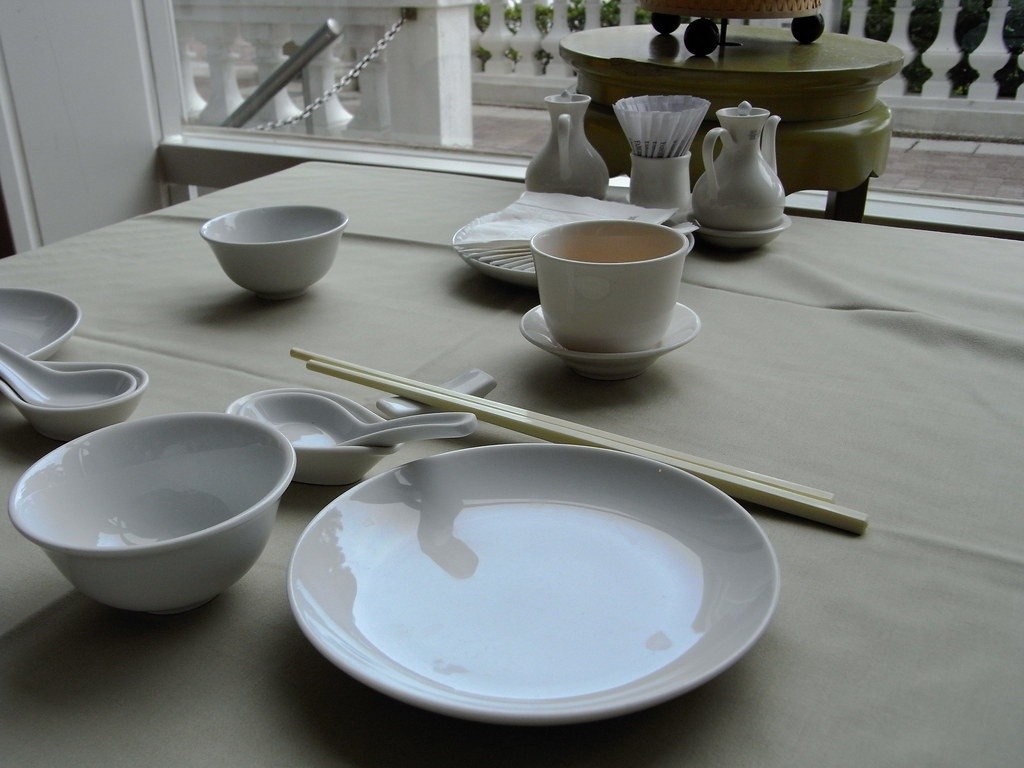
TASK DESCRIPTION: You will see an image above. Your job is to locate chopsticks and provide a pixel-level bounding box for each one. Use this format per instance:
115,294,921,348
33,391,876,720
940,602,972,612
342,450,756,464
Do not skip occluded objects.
290,346,869,536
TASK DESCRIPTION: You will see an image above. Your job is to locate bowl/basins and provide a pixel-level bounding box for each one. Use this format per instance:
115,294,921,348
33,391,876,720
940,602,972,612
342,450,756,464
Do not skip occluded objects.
6,410,298,617
199,205,348,299
0,361,148,442
225,386,407,487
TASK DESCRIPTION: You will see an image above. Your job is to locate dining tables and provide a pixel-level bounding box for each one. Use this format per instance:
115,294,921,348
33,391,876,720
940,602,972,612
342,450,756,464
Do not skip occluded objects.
0,161,1024,767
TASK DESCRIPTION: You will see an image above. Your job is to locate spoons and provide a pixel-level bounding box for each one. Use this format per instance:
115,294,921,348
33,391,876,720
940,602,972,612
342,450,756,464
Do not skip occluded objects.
0,342,136,410
237,395,478,451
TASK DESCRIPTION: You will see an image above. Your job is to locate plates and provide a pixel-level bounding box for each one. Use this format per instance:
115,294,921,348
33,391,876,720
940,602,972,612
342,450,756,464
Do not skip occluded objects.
453,209,695,288
685,215,793,248
285,443,783,723
520,302,701,381
0,286,81,365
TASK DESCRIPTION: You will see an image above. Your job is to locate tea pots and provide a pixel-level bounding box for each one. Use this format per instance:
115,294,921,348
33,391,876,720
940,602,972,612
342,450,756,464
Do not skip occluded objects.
524,90,608,201
692,99,786,231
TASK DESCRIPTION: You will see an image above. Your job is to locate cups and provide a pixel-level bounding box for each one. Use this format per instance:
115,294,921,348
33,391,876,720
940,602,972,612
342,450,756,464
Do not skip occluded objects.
627,152,692,221
529,220,688,355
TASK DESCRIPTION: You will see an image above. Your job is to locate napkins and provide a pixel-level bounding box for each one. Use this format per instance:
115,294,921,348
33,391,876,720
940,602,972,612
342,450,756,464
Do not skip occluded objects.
454,187,701,275
